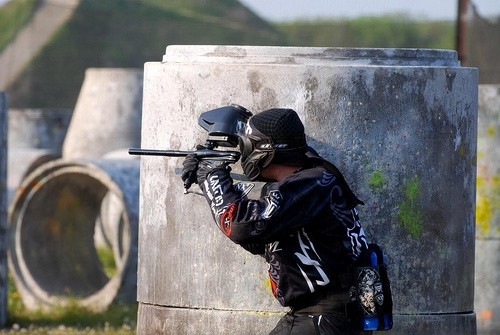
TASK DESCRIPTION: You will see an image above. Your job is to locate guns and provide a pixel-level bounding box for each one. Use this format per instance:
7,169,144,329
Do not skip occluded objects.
128,103,255,200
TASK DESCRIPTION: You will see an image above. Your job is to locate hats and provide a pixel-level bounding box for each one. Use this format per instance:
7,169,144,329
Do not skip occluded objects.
244,109,310,157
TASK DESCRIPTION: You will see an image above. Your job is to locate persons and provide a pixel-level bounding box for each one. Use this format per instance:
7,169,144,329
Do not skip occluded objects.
180,105,395,335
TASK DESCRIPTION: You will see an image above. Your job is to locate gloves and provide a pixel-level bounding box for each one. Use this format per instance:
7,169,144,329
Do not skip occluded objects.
197,160,228,184
181,153,200,183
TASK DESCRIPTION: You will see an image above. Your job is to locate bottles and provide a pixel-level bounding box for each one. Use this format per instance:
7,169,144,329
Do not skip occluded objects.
363,249,393,330
